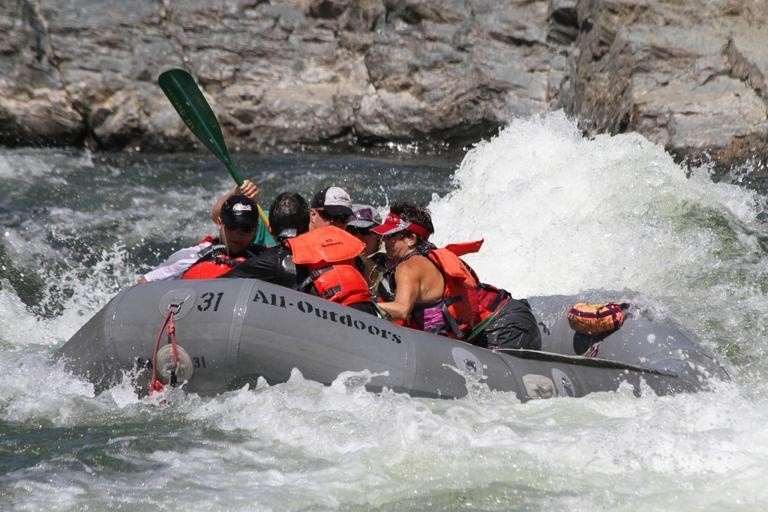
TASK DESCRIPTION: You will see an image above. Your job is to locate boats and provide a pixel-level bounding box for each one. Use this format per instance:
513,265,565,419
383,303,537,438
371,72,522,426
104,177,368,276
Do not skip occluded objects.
49,277,732,410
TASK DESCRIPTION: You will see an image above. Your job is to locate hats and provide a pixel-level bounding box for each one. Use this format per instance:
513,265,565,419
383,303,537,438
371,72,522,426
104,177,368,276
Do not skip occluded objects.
219,194,259,229
311,186,430,240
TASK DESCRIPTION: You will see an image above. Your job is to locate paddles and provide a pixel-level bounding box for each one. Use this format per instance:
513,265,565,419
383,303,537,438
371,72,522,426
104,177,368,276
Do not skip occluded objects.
159,69,270,232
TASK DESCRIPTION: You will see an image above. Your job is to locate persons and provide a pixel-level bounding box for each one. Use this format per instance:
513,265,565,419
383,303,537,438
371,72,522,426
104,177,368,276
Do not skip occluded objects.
209,177,357,250
373,202,541,354
129,193,265,284
345,203,394,303
212,191,376,316
